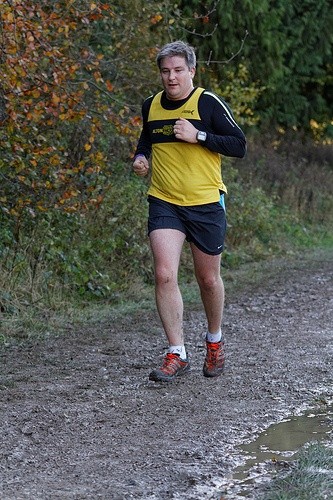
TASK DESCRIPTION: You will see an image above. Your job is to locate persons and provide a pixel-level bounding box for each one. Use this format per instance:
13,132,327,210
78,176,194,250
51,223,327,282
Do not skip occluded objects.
130,39,248,381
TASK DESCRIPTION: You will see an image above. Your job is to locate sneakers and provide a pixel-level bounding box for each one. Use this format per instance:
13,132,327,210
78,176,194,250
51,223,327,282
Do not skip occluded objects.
203,334,225,377
149,352,191,382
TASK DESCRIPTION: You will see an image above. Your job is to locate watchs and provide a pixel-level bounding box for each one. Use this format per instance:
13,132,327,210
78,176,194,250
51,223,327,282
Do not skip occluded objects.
196,130,207,144
